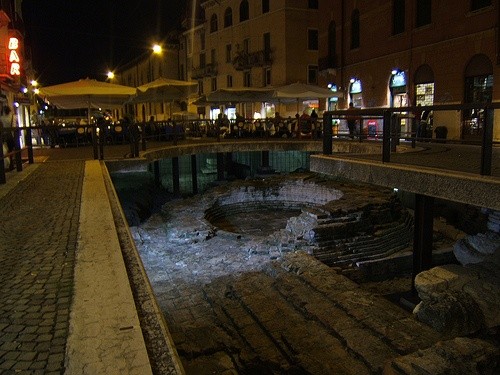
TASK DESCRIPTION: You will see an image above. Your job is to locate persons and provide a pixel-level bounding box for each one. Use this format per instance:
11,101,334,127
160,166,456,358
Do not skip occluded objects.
346,102,357,139
0,105,18,171
40,110,318,148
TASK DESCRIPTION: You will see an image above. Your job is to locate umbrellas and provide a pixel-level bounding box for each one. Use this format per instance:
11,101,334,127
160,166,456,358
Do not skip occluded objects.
136,76,200,120
37,78,137,124
268,81,338,117
207,87,275,119
